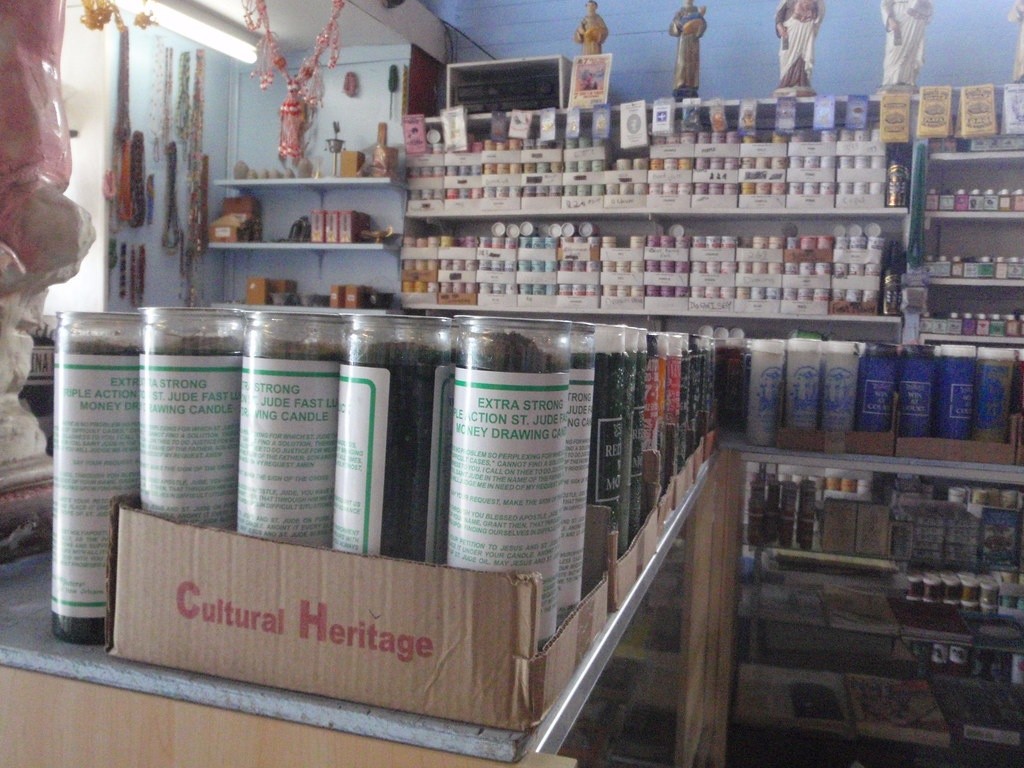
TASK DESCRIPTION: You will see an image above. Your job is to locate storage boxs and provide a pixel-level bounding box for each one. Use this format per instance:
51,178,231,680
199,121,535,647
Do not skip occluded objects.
101,419,718,735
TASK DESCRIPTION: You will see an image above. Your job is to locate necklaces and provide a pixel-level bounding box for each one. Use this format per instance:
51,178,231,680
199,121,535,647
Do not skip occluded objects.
108,24,212,309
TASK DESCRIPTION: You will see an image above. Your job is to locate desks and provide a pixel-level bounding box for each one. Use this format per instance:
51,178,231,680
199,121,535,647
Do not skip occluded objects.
0,431,742,766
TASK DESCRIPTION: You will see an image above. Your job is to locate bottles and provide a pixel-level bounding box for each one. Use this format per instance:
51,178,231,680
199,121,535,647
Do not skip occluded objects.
923,254,1024,279
49,307,1024,678
924,189,1024,211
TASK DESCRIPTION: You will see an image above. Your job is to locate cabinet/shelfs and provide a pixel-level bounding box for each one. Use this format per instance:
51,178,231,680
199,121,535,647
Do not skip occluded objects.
398,82,1023,766
203,176,406,311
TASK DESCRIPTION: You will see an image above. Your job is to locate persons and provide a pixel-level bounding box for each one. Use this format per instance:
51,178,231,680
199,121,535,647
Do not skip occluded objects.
878,0,934,91
668,0,707,101
573,0,609,56
1008,0,1024,85
773,0,823,98
